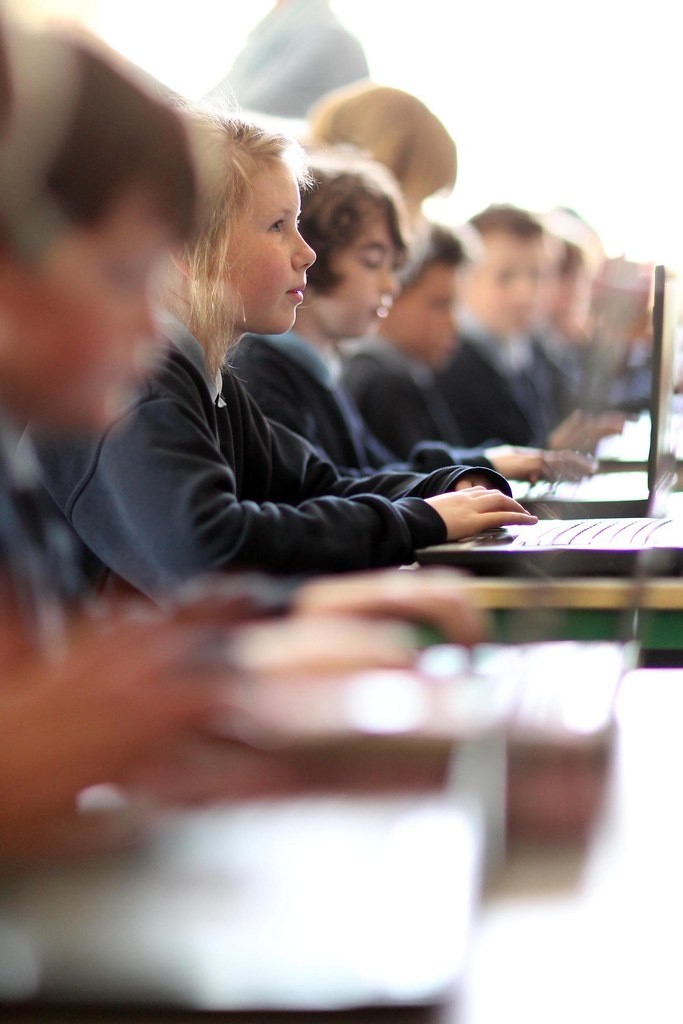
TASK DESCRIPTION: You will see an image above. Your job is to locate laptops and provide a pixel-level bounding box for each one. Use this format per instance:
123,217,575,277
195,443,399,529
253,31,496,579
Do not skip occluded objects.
411,265,683,581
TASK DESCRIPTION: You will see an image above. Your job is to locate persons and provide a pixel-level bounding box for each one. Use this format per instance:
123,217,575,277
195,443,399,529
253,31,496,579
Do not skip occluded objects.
0,1,648,893
